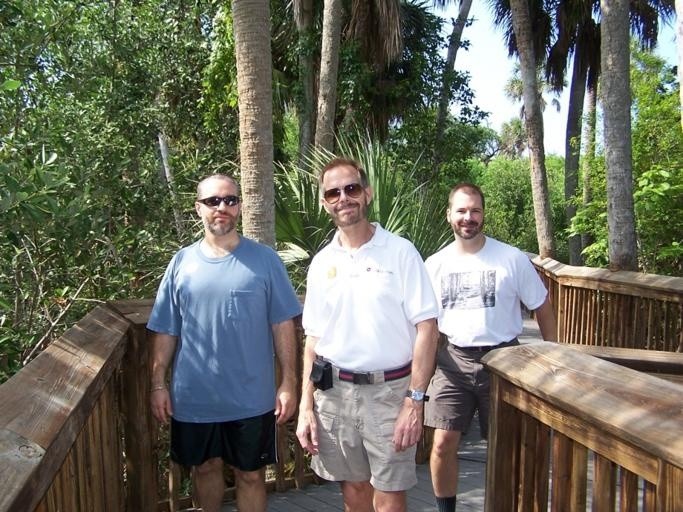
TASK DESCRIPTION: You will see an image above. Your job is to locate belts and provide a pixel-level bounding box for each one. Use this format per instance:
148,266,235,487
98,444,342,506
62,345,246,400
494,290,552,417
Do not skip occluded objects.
452,343,503,353
339,363,410,383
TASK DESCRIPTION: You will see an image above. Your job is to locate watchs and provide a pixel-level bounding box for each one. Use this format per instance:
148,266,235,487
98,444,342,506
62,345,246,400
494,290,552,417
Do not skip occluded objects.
404,389,429,402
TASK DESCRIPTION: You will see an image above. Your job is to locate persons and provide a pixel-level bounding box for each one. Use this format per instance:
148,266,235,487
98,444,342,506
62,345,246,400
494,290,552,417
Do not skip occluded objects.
296,158,439,512
424,183,558,512
146,174,303,512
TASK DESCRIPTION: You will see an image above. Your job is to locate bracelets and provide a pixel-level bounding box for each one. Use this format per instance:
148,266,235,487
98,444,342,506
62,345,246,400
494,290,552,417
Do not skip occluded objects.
149,383,167,393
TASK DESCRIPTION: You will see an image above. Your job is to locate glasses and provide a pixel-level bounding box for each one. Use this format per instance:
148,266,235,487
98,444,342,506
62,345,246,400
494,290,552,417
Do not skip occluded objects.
322,183,365,205
197,196,240,207
